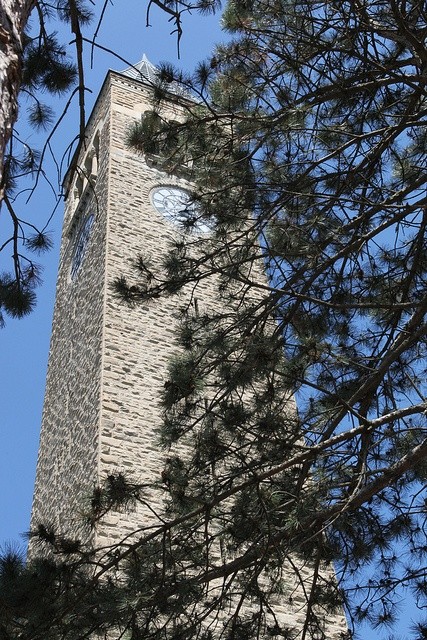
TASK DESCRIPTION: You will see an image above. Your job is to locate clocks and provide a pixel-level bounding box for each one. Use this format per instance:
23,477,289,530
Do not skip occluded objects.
68,215,95,283
148,183,224,234
